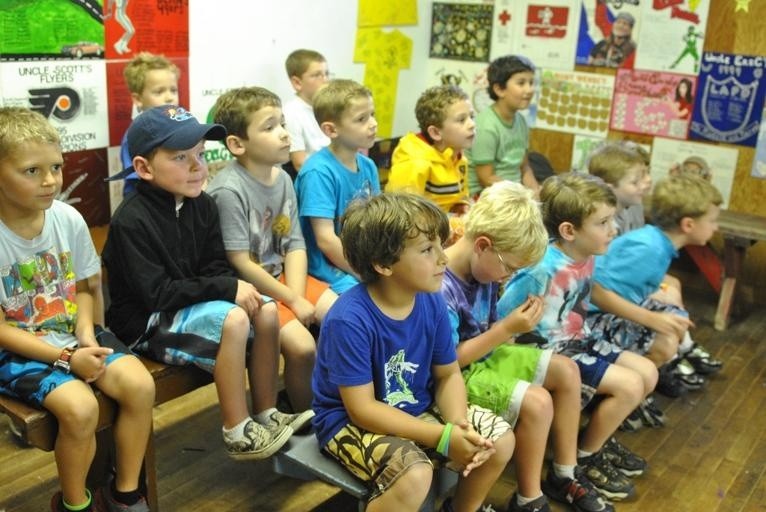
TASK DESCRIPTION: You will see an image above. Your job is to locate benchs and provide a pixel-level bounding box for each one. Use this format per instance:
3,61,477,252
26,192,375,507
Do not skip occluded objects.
1,202,685,512
639,193,764,333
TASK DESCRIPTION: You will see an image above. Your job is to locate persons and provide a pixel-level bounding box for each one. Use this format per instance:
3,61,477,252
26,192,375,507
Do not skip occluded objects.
310,191,516,511
291,76,385,299
441,143,723,511
97,104,317,464
588,12,636,67
118,49,183,208
670,24,706,72
275,51,338,186
203,85,342,414
0,105,157,511
382,81,480,247
660,74,694,140
460,54,549,205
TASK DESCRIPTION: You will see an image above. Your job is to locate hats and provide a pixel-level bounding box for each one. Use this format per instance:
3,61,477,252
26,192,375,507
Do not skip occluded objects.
127,105,228,161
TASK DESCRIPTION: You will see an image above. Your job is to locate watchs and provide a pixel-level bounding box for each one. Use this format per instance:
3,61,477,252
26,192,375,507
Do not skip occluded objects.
52,346,74,373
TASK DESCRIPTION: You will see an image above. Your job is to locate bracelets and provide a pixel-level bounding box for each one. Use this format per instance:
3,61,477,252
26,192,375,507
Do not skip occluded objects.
436,422,454,454
441,431,451,457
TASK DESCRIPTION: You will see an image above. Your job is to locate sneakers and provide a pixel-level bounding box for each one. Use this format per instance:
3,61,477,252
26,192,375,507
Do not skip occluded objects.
507,343,724,512
222,420,294,461
51,491,98,512
254,410,317,436
95,486,151,512
